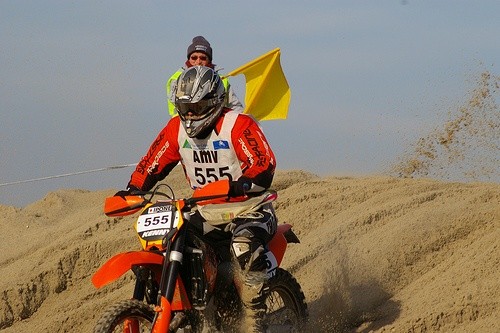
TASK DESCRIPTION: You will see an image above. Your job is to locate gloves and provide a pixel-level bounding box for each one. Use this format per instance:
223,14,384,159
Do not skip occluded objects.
229,175,252,197
113,191,130,200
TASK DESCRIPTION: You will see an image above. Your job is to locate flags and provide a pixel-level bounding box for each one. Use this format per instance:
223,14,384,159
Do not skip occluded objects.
229,48,292,121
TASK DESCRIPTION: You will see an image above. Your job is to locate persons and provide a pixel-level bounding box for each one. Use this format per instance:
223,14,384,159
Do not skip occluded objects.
166,36,243,119
113,66,276,333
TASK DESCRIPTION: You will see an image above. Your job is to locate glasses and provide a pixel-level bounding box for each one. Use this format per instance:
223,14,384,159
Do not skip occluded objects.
190,56,208,61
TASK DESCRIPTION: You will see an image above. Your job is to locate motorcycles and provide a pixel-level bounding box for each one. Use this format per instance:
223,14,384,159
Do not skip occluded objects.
91,179,308,333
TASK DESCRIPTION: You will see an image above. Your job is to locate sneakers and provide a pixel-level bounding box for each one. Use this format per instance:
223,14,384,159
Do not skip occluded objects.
249,311,269,333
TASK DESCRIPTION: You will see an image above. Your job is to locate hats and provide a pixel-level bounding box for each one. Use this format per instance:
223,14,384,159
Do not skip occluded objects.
187,35,212,60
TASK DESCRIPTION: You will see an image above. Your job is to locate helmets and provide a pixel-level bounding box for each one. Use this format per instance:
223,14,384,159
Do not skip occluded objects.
173,66,228,138
